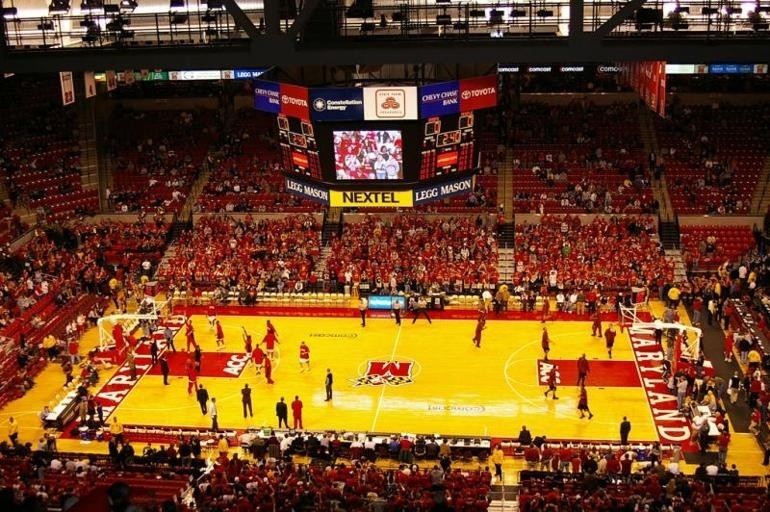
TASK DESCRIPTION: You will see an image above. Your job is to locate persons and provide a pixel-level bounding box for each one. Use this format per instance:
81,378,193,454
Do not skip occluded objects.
0,96,768,511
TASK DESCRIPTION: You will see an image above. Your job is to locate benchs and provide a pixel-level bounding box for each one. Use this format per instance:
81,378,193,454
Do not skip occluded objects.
0,449,206,512
0,283,109,408
